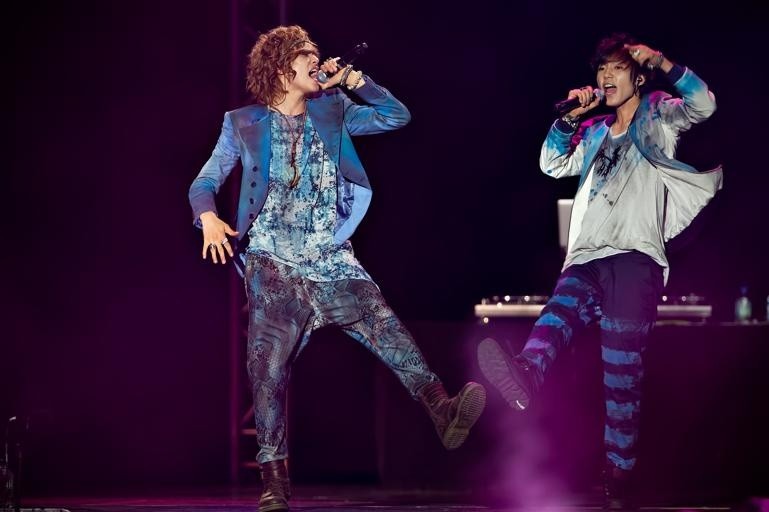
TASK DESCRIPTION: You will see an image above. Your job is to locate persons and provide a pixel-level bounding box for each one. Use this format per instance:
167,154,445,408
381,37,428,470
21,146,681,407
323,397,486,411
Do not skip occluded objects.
185,25,487,512
476,30,724,512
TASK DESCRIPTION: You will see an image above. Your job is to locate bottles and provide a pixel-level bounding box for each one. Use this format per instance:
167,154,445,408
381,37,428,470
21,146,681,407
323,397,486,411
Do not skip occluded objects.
733,286,752,325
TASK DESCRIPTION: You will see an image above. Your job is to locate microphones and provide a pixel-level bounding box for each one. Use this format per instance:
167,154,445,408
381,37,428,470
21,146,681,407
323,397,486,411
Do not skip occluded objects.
317,42,369,83
555,89,605,112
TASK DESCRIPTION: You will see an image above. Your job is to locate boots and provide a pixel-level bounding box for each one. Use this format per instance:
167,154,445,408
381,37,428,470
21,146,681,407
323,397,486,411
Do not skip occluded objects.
418,380,487,451
478,338,536,411
601,458,638,511
260,460,290,512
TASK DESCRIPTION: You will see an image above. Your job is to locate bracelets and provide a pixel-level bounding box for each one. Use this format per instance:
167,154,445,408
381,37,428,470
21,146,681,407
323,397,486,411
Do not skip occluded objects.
340,64,354,86
562,113,580,131
346,70,363,91
646,50,663,70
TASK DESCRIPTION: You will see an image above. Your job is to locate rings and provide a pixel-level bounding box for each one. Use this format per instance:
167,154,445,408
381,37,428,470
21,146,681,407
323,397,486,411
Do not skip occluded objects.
630,47,640,57
222,238,228,244
209,244,217,248
579,87,587,91
